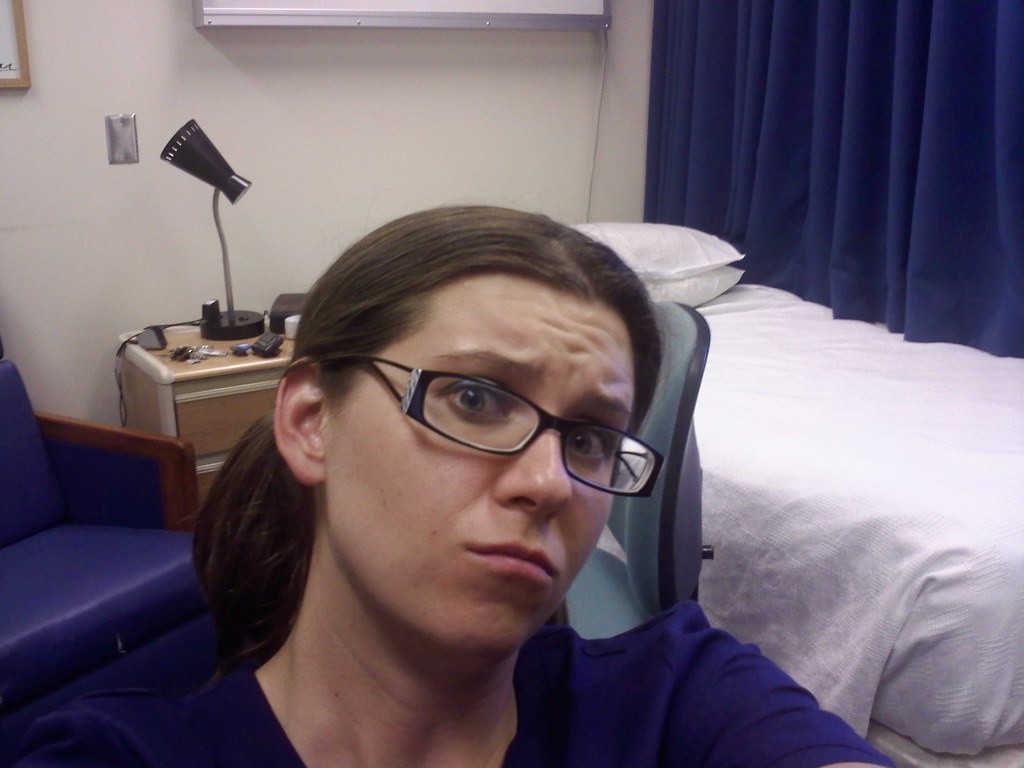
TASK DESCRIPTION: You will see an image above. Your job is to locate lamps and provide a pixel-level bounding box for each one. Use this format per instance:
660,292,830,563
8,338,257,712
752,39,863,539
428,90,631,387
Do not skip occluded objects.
159,118,265,343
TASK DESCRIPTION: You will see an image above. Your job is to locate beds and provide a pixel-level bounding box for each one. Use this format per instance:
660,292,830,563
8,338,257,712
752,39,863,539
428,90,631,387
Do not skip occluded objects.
693,283,1023,768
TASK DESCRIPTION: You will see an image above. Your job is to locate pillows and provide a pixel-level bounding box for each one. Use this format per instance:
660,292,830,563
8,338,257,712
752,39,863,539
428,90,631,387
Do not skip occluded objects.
572,222,748,311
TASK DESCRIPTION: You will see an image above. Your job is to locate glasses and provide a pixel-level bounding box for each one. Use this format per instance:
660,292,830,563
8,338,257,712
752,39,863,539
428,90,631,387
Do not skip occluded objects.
320,351,666,499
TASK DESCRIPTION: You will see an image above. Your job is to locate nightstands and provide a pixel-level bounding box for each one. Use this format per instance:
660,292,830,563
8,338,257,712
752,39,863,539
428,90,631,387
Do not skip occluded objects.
118,326,296,506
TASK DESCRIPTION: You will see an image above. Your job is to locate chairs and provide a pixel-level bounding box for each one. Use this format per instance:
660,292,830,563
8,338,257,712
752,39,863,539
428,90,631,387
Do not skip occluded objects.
1,334,199,728
565,299,715,642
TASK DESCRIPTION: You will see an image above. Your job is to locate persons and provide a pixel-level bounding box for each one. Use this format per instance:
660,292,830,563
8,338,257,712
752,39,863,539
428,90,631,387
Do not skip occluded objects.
14,205,897,768
